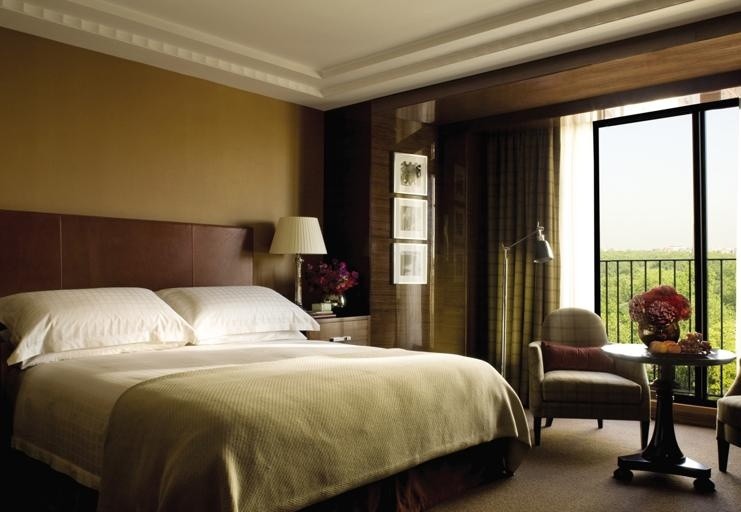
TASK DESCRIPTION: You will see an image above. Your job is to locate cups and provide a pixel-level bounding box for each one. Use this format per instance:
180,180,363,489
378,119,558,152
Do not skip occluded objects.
710,346,722,354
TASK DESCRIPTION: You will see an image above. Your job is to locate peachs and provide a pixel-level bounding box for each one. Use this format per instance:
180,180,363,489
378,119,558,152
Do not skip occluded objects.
649,340,681,355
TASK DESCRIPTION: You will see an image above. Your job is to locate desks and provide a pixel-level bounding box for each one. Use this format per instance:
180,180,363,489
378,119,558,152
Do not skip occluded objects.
599,343,737,491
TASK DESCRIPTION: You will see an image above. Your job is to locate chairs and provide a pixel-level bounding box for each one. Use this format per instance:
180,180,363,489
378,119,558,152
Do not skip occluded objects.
528,307,651,450
716,355,741,471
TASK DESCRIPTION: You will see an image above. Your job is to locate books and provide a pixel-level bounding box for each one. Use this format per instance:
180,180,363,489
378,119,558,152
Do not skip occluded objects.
304,310,337,319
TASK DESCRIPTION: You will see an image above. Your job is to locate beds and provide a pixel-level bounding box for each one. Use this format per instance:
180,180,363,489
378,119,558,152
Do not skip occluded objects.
0,209,533,511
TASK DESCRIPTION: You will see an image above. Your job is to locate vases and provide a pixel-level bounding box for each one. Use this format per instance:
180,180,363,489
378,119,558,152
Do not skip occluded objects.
324,294,347,316
638,322,680,348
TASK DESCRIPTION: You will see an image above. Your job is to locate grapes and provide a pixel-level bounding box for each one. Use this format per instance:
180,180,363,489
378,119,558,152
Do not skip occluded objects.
678,333,712,354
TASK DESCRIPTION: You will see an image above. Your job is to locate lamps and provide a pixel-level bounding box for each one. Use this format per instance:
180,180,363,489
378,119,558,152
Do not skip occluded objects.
268,216,328,319
499,221,554,377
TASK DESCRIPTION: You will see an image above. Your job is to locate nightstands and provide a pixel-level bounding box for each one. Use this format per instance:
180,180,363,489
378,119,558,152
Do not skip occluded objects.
301,316,372,347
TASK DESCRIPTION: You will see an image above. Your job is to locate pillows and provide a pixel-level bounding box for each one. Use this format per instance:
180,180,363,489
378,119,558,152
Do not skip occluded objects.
188,329,309,346
0,328,191,371
541,341,616,373
0,287,196,367
155,285,321,337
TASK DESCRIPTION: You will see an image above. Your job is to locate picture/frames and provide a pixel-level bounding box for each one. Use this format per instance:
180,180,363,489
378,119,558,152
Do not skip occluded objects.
389,151,429,197
391,240,429,285
390,195,429,242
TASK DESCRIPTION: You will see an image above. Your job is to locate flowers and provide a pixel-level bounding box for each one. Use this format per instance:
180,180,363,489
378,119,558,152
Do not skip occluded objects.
303,259,359,296
628,285,692,327
